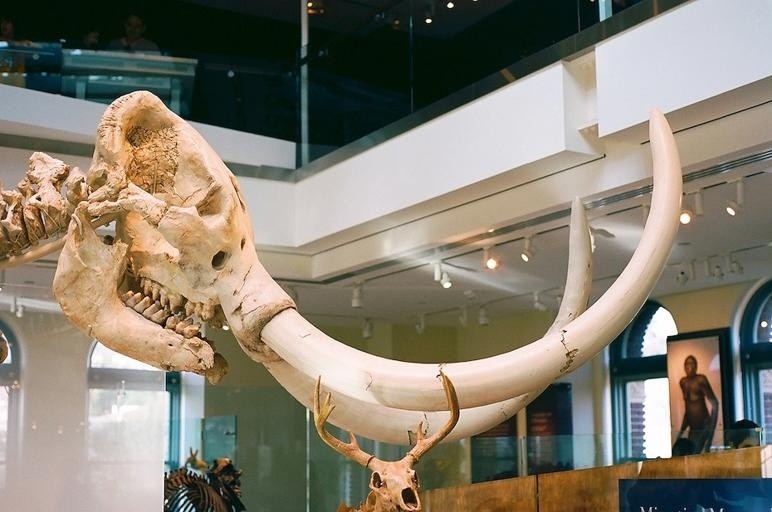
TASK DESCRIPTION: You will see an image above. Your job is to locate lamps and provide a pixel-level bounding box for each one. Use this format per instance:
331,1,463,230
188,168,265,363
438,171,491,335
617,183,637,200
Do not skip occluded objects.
349,281,376,339
458,290,493,327
680,177,745,223
415,308,427,338
10,296,25,318
533,289,568,313
676,252,744,286
433,235,536,289
641,192,653,228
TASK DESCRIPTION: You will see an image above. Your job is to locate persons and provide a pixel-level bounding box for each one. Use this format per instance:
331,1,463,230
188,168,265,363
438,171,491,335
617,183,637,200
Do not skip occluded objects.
675,355,721,453
